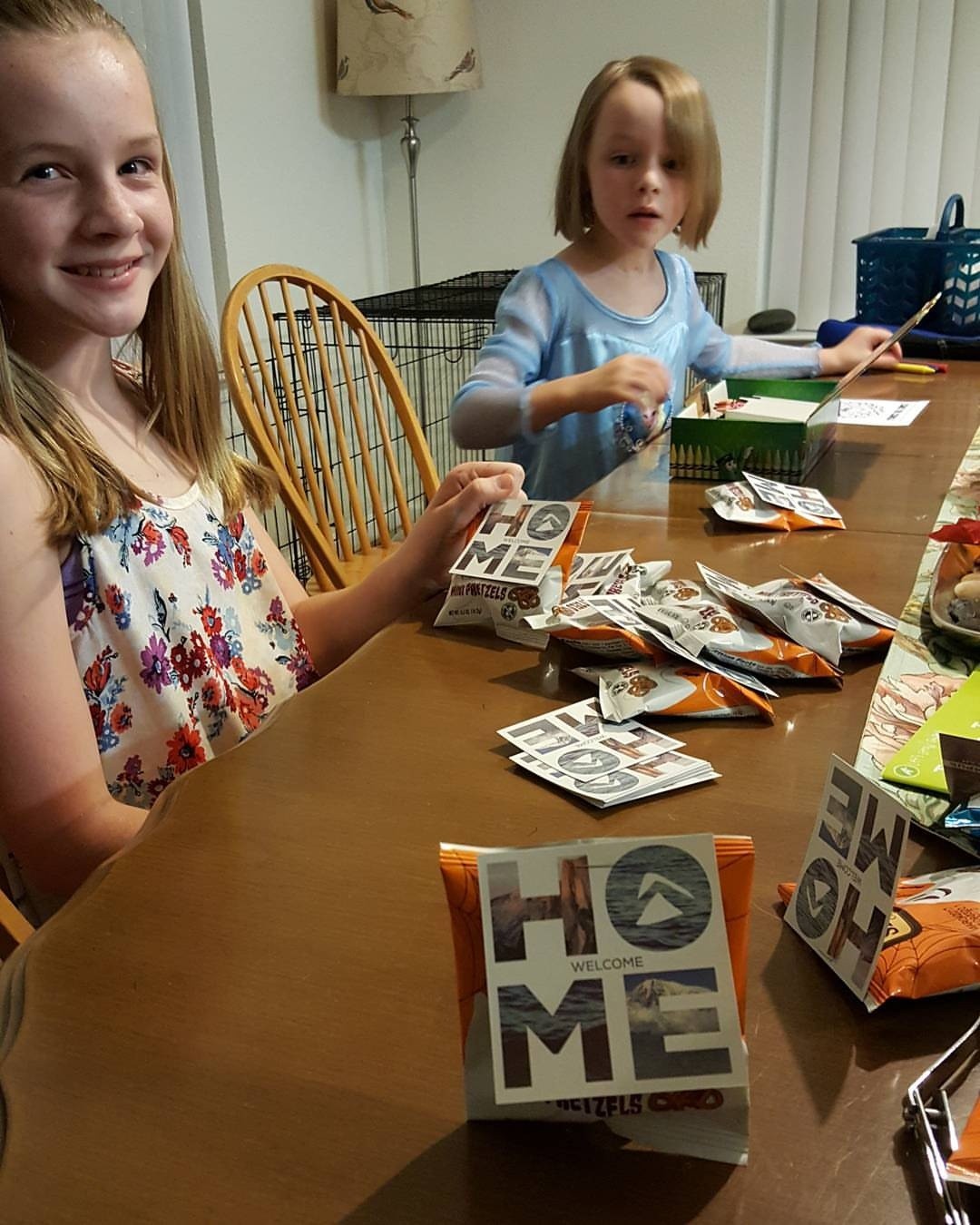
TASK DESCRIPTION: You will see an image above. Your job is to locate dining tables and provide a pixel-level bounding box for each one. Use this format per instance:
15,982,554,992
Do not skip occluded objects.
0,362,980,1225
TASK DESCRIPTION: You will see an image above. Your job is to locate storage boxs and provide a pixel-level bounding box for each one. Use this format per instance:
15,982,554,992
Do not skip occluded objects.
664,292,943,480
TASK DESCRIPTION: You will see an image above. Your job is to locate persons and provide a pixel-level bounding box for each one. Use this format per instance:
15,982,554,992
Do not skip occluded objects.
0,1,527,925
446,55,904,501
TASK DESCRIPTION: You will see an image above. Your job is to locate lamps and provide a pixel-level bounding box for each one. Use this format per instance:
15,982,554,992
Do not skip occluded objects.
334,1,485,513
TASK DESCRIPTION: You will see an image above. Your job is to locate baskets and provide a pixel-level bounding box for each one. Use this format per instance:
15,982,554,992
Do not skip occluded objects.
851,193,980,339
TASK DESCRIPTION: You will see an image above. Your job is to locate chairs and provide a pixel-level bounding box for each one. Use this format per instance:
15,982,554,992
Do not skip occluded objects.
221,263,441,604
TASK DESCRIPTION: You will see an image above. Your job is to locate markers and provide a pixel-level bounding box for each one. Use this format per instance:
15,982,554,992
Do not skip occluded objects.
895,362,936,375
901,361,950,374
